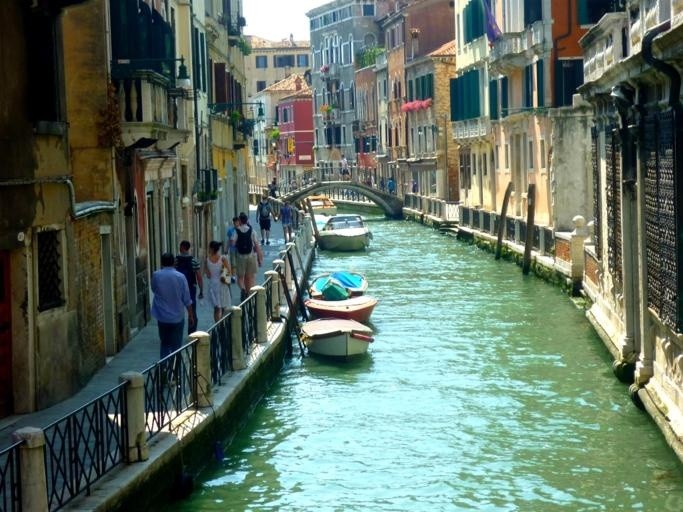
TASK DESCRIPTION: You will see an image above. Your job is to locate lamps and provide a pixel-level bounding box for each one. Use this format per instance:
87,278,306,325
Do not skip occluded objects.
206,99,265,119
108,52,191,91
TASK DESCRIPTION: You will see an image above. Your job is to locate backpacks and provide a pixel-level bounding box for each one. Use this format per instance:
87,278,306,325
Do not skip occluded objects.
259,201,269,218
234,226,253,255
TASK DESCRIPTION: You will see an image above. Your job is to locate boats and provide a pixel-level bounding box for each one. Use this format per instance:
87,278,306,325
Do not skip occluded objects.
308,269,368,299
305,295,379,325
301,318,374,358
300,194,337,216
318,213,372,252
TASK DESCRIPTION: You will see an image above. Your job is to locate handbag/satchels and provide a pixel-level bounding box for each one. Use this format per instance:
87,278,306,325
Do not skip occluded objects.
220,264,231,285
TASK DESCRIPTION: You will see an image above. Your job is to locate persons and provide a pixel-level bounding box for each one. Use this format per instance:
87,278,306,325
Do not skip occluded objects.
172,240,203,335
239,211,253,229
360,171,418,198
223,216,241,283
275,200,293,245
255,193,275,245
336,152,351,183
266,173,316,201
203,240,232,327
150,251,195,388
230,215,262,302
